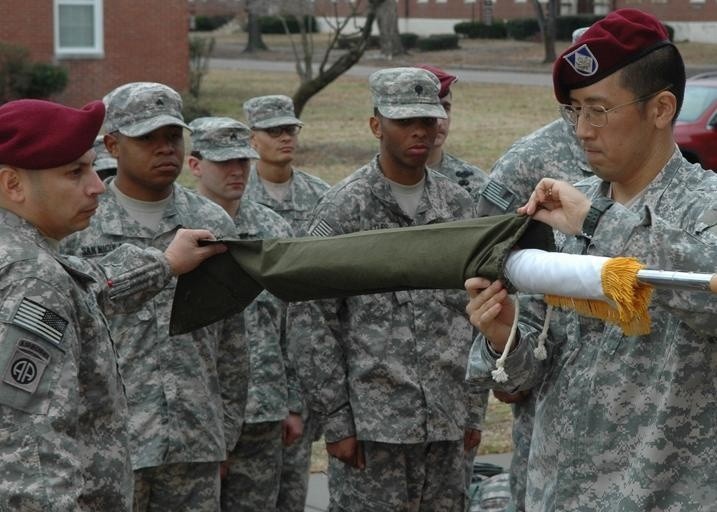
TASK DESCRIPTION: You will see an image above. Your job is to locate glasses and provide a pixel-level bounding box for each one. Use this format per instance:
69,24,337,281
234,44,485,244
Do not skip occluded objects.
253,125,302,137
557,84,672,127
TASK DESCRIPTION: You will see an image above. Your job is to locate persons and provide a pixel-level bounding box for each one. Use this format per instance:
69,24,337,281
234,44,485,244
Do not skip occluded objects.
279,66,492,510
472,23,676,510
237,93,331,443
461,6,716,511
59,81,252,512
414,63,490,491
87,134,118,182
0,98,228,511
186,114,307,511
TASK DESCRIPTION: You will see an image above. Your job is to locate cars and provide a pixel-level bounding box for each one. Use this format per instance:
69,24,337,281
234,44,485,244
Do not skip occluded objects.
671,70,717,173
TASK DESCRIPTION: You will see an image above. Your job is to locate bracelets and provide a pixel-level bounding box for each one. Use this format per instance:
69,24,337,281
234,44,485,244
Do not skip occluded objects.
581,197,613,248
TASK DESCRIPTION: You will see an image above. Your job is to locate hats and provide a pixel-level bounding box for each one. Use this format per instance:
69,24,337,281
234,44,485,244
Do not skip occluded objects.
102,83,194,138
189,118,258,162
244,95,304,128
0,99,105,168
368,65,458,120
93,137,118,171
554,8,675,104
